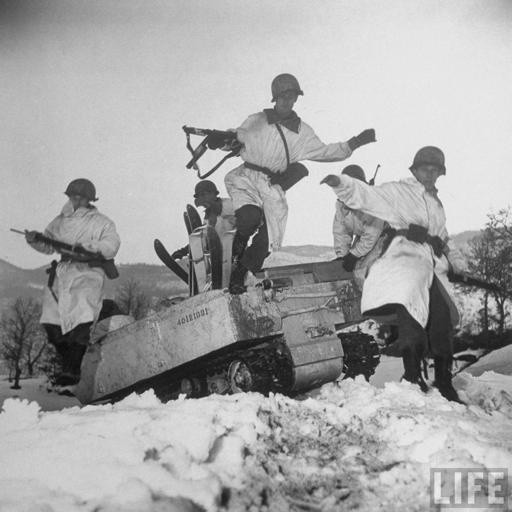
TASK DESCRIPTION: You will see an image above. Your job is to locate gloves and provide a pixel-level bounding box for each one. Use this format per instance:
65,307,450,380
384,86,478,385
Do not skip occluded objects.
343,254,357,272
209,137,225,149
358,128,376,145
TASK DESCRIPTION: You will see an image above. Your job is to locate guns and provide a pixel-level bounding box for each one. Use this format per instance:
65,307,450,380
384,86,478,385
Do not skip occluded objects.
353,164,382,250
449,270,499,300
181,126,243,169
9,228,119,278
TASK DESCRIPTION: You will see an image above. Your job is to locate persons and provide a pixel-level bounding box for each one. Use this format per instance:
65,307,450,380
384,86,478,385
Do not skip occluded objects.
24,177,122,388
206,73,377,294
332,162,394,293
317,146,471,406
171,180,240,289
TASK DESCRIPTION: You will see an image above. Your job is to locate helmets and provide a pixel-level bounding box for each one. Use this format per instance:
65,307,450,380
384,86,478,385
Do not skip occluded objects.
271,74,303,103
193,180,219,199
409,147,445,173
342,165,366,184
66,180,98,202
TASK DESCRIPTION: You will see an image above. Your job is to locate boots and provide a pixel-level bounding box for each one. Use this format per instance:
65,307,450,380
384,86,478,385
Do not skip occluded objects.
435,359,458,401
401,346,428,392
228,231,250,294
57,341,86,386
